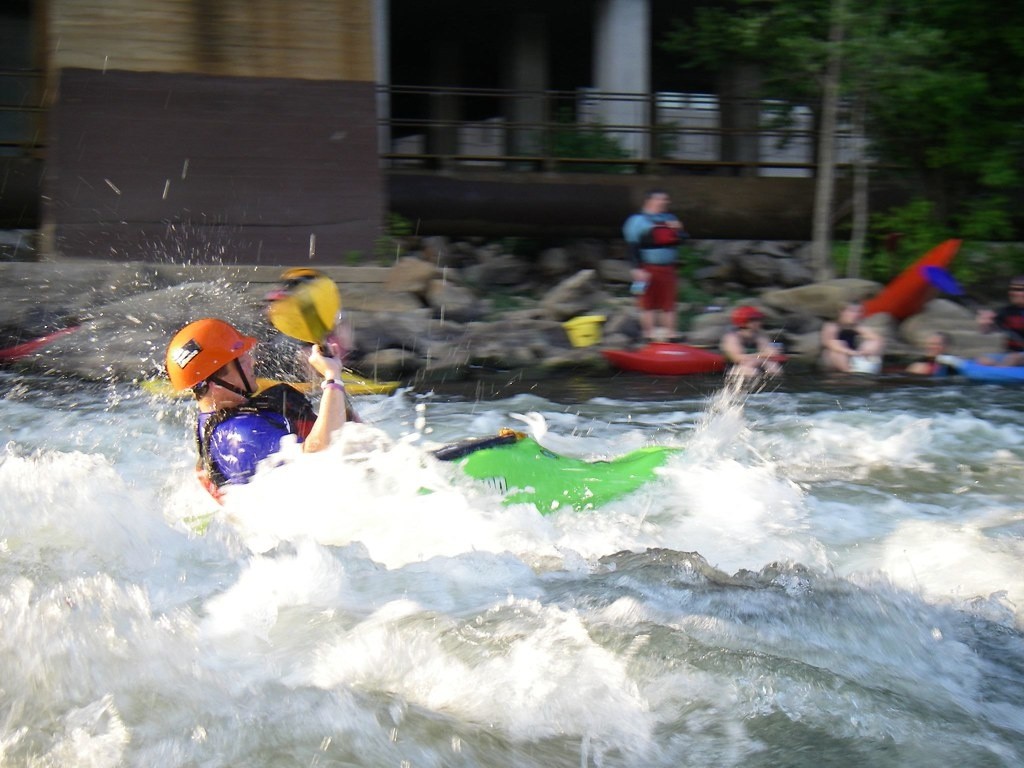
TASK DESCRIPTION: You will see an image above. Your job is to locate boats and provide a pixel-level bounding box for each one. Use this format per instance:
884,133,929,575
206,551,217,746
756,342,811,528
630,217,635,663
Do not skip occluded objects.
141,373,403,401
860,236,965,322
935,354,1023,386
594,341,788,375
0,322,77,366
418,430,689,515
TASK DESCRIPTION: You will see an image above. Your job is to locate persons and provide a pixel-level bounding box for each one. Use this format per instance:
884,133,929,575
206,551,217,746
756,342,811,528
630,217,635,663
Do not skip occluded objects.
818,298,884,377
720,305,789,394
905,331,957,376
623,190,688,339
166,317,362,489
976,275,1024,368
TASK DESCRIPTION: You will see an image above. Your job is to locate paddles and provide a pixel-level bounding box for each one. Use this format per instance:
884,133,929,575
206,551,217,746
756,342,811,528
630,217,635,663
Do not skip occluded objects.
263,266,362,425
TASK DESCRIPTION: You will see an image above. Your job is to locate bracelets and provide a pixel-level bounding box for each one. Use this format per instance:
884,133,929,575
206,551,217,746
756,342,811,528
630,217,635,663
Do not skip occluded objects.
321,379,345,394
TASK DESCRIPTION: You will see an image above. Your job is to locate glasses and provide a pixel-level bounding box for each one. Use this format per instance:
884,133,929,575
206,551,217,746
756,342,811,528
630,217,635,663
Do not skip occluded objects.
1007,287,1024,292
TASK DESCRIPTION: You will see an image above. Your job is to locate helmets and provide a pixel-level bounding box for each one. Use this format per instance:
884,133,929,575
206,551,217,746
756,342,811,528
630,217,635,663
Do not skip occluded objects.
733,306,767,327
283,268,321,285
166,318,257,391
